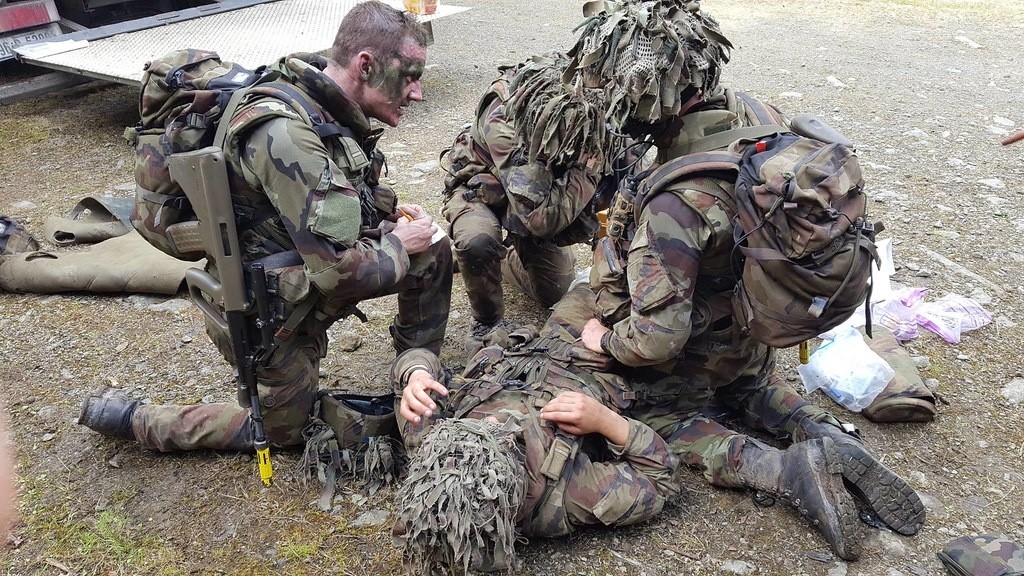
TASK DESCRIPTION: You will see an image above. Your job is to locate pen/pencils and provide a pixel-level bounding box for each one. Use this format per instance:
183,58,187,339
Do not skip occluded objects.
397,208,413,221
827,385,841,398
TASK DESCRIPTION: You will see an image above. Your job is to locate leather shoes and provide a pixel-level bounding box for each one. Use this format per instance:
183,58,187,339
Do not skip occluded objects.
77,386,134,437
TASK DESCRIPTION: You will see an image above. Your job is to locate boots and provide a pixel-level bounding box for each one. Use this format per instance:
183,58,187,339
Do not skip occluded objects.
799,417,926,534
737,438,859,560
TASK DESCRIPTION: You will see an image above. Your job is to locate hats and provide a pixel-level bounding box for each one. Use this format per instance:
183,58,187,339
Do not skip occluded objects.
509,51,626,170
561,0,731,120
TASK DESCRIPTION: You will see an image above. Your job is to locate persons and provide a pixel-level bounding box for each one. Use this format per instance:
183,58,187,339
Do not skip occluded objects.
390,268,680,576
78,1,454,450
440,50,643,352
581,0,927,562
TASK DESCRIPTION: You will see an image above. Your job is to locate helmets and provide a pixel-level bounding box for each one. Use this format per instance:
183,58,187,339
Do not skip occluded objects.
318,389,395,451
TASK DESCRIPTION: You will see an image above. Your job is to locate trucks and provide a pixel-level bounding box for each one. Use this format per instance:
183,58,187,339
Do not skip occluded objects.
0,0,474,88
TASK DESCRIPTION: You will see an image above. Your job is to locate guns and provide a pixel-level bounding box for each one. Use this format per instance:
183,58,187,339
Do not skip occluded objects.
165,145,278,489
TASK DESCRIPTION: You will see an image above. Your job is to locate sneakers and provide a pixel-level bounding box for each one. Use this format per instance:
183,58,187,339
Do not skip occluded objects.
501,245,520,289
465,316,505,356
452,250,459,274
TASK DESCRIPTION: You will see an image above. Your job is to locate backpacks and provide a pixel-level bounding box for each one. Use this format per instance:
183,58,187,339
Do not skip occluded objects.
635,92,885,348
130,48,333,262
